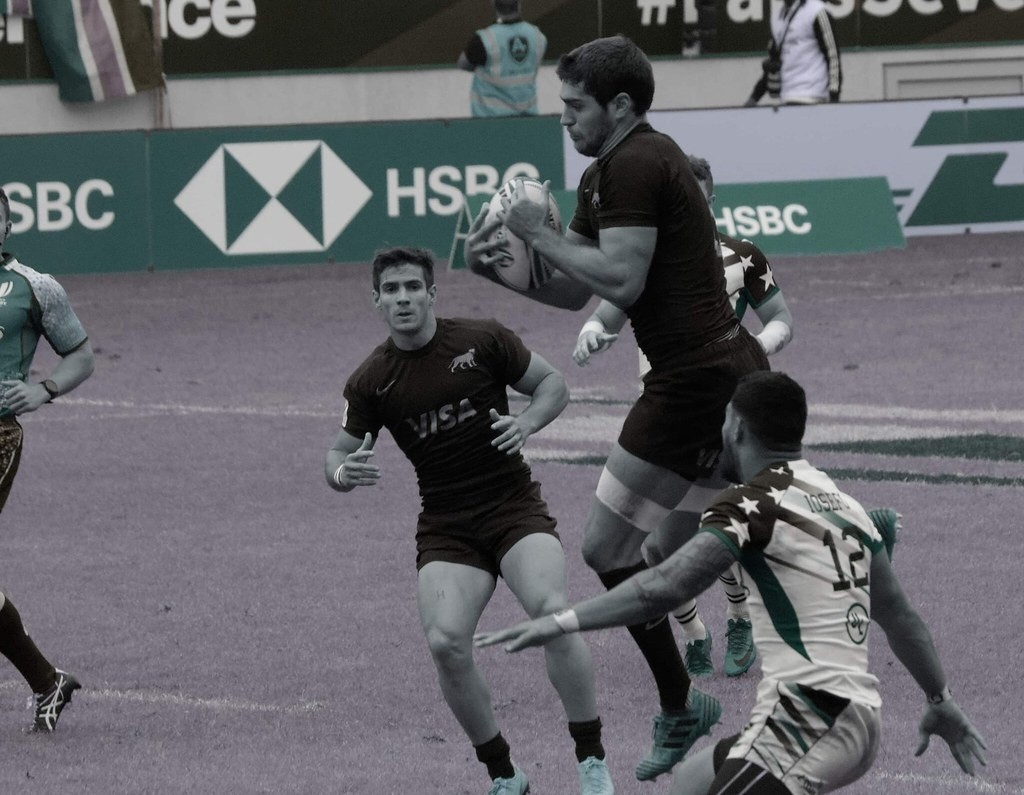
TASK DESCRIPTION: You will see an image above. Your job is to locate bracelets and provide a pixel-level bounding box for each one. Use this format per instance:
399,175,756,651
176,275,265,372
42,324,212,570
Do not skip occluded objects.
927,684,951,705
553,608,580,633
334,464,344,486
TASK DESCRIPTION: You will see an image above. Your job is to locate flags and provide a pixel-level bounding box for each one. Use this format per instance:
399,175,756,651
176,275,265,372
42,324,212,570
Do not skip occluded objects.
34,0,164,100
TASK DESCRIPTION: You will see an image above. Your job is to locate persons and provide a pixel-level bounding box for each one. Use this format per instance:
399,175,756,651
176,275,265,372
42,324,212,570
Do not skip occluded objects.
463,37,771,783
473,369,988,795
324,246,616,795
767,0,842,104
0,188,96,736
573,154,794,676
458,0,547,118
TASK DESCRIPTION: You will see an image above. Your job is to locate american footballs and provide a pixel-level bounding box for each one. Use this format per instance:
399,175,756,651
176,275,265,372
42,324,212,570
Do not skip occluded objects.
483,174,564,294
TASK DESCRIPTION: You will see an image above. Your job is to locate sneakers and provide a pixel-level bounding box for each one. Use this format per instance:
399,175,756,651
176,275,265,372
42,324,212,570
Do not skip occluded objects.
488,759,530,795
684,625,714,680
30,668,82,734
635,687,722,782
870,509,904,565
724,616,757,677
573,746,614,795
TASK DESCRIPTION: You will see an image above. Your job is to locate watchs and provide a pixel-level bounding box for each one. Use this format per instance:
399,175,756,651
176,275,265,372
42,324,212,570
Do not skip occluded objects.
39,379,58,404
